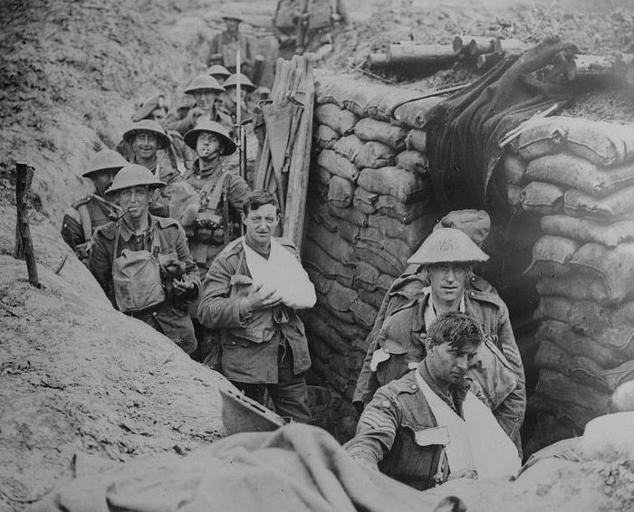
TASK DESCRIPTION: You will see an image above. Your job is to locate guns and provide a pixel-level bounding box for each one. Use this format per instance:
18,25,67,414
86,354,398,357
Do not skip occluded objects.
239,128,247,236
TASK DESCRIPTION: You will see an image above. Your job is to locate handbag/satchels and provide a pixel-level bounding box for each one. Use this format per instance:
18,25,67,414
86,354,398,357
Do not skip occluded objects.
458,331,523,417
222,274,282,345
112,247,167,311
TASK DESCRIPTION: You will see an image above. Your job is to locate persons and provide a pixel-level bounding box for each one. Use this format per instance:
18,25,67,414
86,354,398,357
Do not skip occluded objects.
340,310,521,490
194,191,317,424
361,227,526,461
294,0,340,56
382,268,500,319
64,65,262,357
349,207,491,414
268,1,307,59
207,8,261,83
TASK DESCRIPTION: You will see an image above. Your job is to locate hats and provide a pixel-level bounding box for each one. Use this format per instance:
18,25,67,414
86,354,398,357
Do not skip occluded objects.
131,95,169,122
182,118,237,157
407,226,489,265
121,119,173,151
103,163,168,193
83,146,127,174
185,63,255,95
